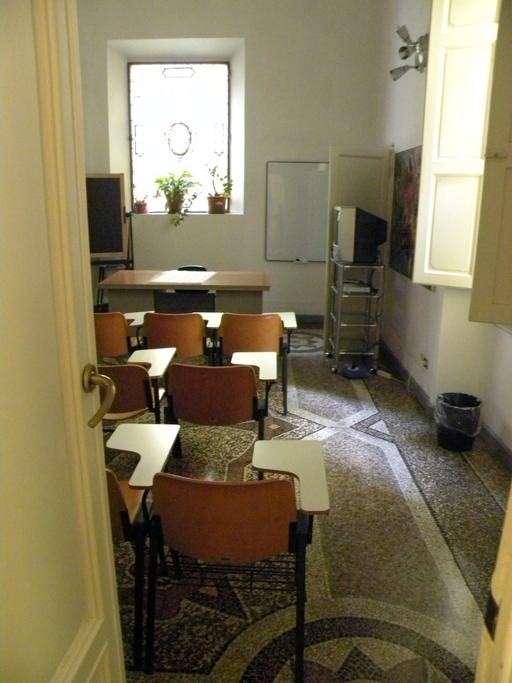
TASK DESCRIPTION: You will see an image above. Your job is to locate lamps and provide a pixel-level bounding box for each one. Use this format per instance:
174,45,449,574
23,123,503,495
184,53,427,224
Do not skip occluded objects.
390,24,429,81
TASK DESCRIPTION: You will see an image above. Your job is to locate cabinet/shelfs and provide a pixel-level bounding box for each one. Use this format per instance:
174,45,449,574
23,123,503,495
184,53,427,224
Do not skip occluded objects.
325,259,387,374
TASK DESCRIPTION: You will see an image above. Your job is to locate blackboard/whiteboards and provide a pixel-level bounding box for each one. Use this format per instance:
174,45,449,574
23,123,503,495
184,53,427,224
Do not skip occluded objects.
265,161,328,262
85,173,128,261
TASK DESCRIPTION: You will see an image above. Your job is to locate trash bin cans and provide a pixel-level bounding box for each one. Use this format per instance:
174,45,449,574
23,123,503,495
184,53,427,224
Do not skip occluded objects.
435,392,483,452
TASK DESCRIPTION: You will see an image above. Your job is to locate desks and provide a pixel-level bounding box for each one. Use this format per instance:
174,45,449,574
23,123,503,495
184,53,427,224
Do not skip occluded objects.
231,351,278,416
127,347,177,408
252,440,329,544
97,269,271,314
106,423,181,523
123,310,298,353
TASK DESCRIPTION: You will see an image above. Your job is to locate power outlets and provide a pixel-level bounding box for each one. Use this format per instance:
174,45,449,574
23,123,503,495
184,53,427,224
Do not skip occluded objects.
418,353,429,370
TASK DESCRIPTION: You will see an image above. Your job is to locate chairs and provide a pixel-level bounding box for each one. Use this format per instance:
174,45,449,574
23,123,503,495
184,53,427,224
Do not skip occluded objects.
163,364,266,459
97,365,160,433
145,473,306,683
173,265,209,295
142,313,206,358
105,468,153,672
93,313,137,359
217,312,288,416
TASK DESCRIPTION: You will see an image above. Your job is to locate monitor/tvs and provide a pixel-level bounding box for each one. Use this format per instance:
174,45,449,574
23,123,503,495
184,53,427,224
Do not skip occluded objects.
332,205,389,265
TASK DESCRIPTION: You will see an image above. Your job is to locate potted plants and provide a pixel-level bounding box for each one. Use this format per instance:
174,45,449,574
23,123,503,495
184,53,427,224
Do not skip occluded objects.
206,165,233,214
152,170,204,227
134,195,148,213
